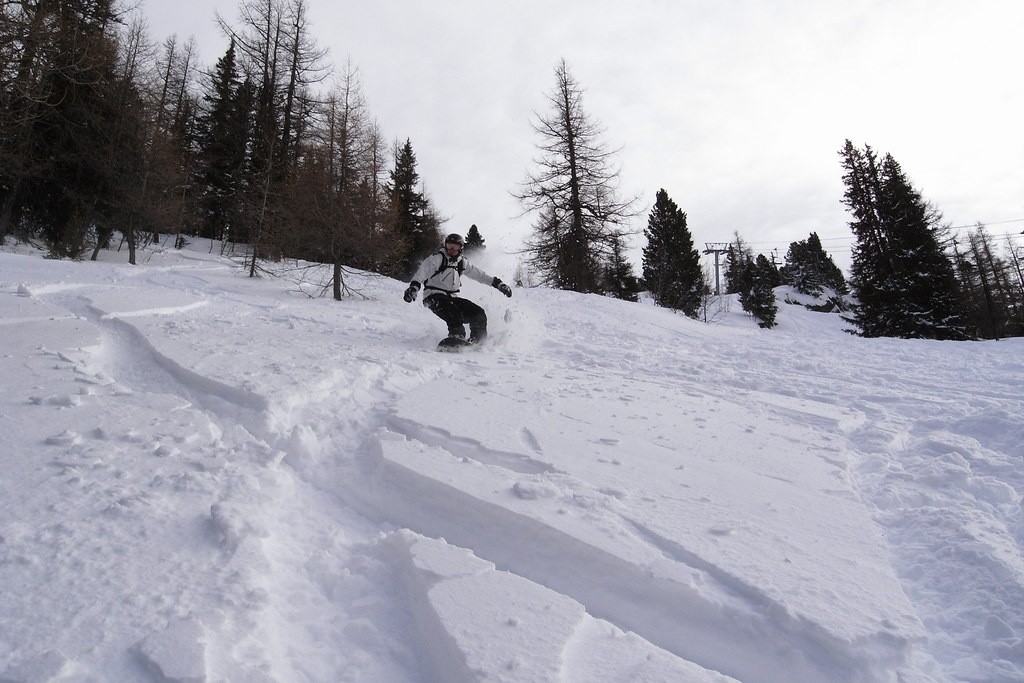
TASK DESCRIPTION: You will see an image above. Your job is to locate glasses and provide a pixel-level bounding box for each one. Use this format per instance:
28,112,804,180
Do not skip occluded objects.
446,243,461,251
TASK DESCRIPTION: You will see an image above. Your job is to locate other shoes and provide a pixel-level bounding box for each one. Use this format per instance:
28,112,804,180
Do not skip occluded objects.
468,336,481,344
448,332,466,340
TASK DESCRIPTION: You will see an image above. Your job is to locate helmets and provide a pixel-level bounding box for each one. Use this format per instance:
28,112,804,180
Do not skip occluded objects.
445,233,464,252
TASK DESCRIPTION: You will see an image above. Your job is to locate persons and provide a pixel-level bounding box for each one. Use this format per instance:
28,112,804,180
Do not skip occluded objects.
404,234,512,345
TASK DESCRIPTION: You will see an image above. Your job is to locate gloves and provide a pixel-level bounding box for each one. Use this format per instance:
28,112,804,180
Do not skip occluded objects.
492,277,512,297
404,281,421,302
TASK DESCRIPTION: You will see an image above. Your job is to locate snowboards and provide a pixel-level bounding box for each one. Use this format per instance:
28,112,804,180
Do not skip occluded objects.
437,336,476,349
